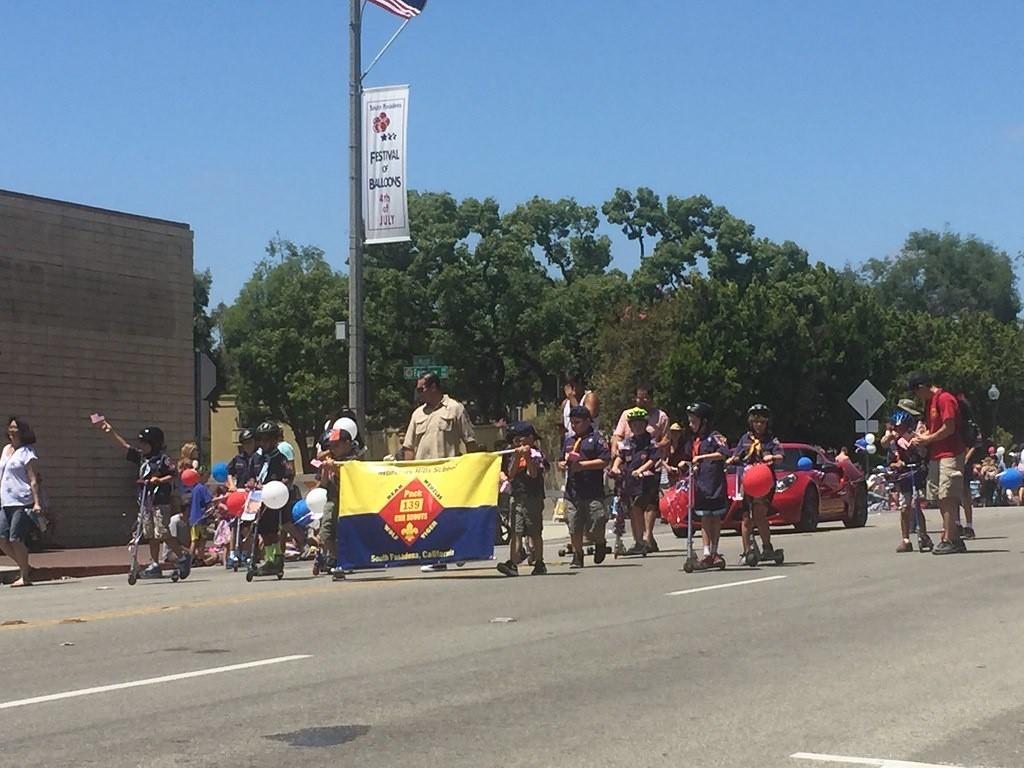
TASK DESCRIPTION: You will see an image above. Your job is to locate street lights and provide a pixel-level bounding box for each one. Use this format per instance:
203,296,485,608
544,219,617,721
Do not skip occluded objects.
988,384,999,447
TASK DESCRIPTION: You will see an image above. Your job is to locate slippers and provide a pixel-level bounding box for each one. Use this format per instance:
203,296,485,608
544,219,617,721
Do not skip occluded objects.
10,578,33,586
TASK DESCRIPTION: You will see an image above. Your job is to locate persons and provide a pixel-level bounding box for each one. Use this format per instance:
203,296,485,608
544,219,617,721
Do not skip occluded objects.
103,372,1024,583
0,416,45,586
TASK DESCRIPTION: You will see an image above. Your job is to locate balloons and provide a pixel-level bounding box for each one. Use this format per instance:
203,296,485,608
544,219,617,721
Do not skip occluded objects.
742,464,773,498
181,469,200,486
306,487,328,514
865,433,875,444
798,456,813,472
262,480,289,509
866,444,876,454
292,500,313,526
989,447,994,454
660,491,687,523
997,447,1005,456
212,463,228,483
226,492,248,517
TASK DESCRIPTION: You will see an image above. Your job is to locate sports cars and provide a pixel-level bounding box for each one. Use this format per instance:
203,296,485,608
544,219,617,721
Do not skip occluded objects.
659,443,866,538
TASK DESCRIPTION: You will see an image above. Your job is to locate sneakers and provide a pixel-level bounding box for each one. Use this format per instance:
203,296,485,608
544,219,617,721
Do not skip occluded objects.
497,560,519,576
420,564,447,571
531,562,547,574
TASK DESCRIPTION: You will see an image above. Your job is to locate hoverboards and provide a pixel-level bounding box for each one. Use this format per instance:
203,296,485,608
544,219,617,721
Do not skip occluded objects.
733,458,786,568
899,458,934,553
684,461,726,572
609,472,650,560
312,548,334,575
127,477,181,585
224,488,285,581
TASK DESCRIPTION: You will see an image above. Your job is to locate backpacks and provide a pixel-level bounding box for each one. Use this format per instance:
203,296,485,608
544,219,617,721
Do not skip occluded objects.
926,390,977,446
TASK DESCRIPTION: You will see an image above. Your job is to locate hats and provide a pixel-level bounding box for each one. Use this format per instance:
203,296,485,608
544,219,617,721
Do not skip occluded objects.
514,422,545,440
669,422,681,431
866,480,875,490
324,428,352,445
898,399,921,415
568,406,595,424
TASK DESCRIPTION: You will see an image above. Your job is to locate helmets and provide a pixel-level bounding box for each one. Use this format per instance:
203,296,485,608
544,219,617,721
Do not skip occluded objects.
138,428,164,443
746,404,769,417
254,421,279,434
626,407,649,421
686,402,714,420
239,427,257,443
890,411,913,427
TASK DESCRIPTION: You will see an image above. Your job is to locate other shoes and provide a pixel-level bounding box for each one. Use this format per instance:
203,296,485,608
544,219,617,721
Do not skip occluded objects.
896,541,913,552
569,550,585,567
594,539,607,564
697,555,714,566
141,565,162,576
178,553,192,579
711,552,724,566
762,543,774,556
917,535,935,552
628,539,659,554
932,538,967,553
957,525,975,540
259,554,284,570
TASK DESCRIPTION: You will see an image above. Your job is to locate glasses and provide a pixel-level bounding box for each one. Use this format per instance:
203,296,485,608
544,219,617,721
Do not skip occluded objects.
636,397,648,403
417,385,432,393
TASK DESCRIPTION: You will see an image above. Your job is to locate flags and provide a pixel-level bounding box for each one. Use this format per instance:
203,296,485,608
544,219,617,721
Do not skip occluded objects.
368,0,427,21
